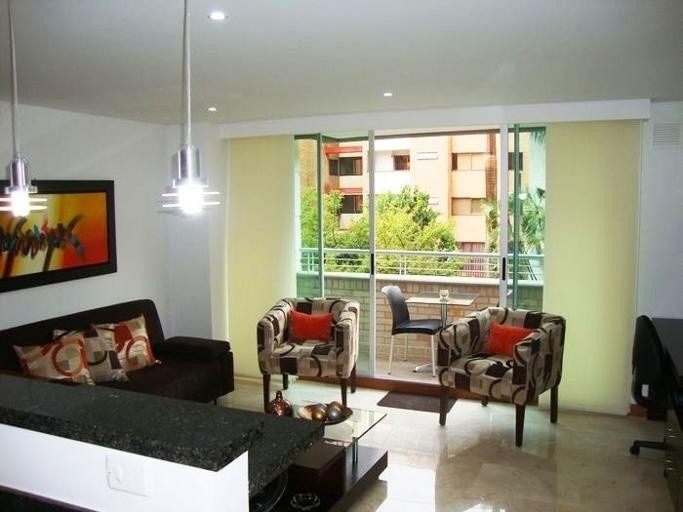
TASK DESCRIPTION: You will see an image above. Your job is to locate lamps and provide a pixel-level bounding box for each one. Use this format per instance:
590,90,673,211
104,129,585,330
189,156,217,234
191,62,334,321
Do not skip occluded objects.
0,1,50,219
158,1,224,219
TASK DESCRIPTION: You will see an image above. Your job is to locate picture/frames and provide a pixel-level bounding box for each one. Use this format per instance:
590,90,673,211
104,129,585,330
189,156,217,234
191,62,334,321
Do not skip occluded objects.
0,178,119,295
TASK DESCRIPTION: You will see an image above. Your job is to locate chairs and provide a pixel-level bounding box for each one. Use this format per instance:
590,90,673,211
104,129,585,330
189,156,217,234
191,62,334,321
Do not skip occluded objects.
256,294,362,409
381,285,445,379
434,303,567,448
625,313,669,478
495,287,514,310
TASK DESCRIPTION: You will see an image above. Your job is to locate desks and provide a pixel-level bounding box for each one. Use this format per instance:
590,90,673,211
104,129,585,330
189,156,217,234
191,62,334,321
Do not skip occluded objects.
649,314,681,512
289,403,390,512
402,289,478,374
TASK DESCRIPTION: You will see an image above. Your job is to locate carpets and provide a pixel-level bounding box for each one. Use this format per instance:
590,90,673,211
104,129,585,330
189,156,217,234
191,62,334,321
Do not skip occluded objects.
374,387,458,415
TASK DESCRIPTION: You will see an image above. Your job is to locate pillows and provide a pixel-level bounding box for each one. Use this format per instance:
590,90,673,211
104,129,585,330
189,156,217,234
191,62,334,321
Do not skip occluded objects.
478,320,537,359
111,312,163,373
283,306,334,344
51,319,131,384
9,328,95,386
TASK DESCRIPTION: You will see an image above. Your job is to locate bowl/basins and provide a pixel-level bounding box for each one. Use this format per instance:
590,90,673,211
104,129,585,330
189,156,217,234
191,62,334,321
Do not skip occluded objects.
298,404,353,424
290,492,320,510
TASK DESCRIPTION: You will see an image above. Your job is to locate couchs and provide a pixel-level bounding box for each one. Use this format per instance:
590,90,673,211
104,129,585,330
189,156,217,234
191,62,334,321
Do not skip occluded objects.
0,296,235,405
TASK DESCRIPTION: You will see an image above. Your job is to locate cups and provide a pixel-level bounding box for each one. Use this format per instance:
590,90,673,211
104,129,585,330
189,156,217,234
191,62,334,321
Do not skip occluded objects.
439,289,448,302
